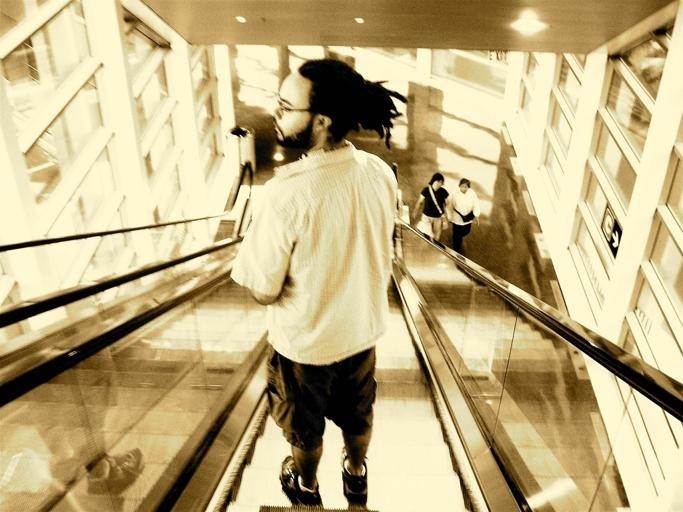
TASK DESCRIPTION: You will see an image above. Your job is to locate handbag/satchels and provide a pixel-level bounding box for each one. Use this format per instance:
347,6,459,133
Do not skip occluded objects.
461,211,474,223
441,216,449,232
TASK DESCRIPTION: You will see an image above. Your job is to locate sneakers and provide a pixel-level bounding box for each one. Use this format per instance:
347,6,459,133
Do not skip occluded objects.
338,445,367,505
87,448,143,496
279,454,322,506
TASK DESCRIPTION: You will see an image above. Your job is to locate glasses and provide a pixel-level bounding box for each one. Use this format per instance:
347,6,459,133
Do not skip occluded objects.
276,101,311,117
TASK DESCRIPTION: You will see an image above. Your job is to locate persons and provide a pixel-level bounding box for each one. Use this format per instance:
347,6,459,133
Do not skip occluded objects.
410,173,449,241
445,178,480,255
229,59,409,505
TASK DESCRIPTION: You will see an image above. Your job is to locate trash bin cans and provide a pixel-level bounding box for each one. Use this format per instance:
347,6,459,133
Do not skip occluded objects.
224,128,239,176
239,126,257,175
